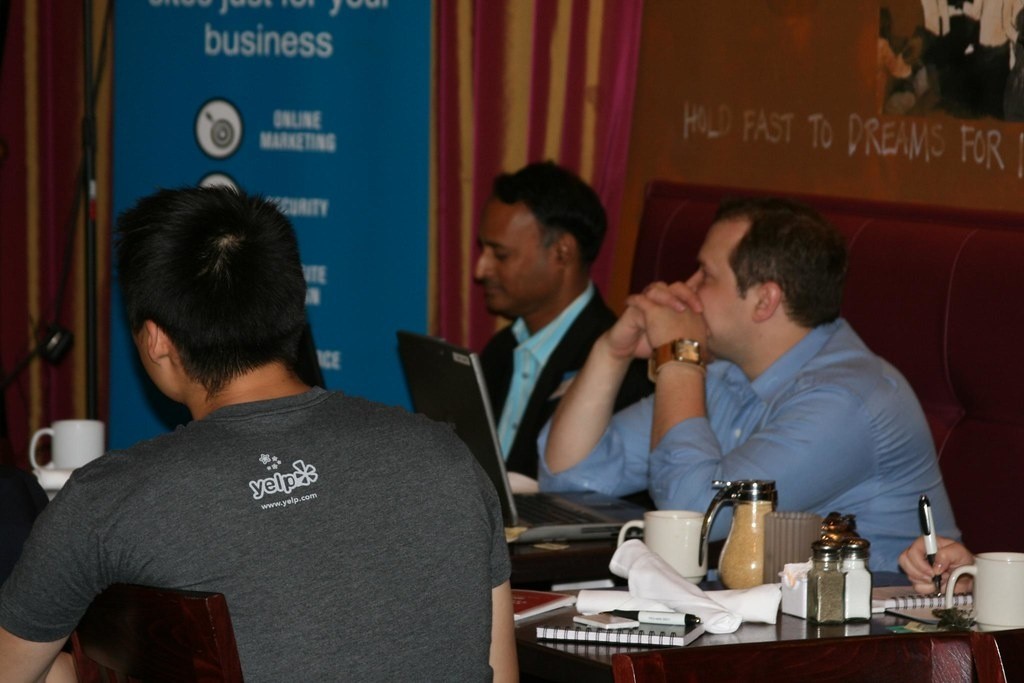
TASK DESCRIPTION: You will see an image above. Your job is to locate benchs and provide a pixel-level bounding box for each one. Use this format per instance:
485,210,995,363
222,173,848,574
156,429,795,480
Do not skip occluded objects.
621,176,1024,553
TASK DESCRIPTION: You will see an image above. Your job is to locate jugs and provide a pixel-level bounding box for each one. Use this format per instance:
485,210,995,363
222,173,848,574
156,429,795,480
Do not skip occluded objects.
698,479,779,590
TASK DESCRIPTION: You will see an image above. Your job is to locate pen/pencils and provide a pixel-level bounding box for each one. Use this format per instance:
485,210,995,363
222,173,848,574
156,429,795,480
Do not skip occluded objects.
918,495,942,598
599,609,700,625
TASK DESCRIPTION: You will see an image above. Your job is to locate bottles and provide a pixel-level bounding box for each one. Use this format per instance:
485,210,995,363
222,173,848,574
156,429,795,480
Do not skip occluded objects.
839,537,874,623
807,539,845,625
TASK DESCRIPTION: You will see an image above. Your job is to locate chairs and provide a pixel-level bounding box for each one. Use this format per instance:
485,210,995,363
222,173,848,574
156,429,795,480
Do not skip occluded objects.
66,580,245,683
610,630,1006,683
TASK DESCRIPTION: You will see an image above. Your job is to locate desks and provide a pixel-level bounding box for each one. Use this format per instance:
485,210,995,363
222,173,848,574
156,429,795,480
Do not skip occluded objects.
505,539,614,577
509,586,1024,683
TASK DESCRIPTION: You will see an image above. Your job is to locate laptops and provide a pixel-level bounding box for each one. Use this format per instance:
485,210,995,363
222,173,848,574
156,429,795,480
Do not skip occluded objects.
394,329,659,541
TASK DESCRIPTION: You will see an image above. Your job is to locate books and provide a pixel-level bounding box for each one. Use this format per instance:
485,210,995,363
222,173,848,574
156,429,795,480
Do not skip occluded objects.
512,589,705,646
871,586,974,624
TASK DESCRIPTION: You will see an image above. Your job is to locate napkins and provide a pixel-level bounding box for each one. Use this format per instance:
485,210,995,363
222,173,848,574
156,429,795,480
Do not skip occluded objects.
575,538,782,635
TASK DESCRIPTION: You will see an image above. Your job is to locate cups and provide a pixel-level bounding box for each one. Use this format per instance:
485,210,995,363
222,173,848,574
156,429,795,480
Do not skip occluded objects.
29,419,104,472
945,552,1024,626
762,512,822,584
617,509,708,585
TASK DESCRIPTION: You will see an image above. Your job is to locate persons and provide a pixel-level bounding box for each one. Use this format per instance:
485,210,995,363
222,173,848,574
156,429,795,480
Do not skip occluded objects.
537,194,965,583
473,160,655,479
0,184,519,683
897,533,973,596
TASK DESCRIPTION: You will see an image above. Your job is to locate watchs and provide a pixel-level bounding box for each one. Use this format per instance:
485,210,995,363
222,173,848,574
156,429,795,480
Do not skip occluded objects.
648,337,709,383
